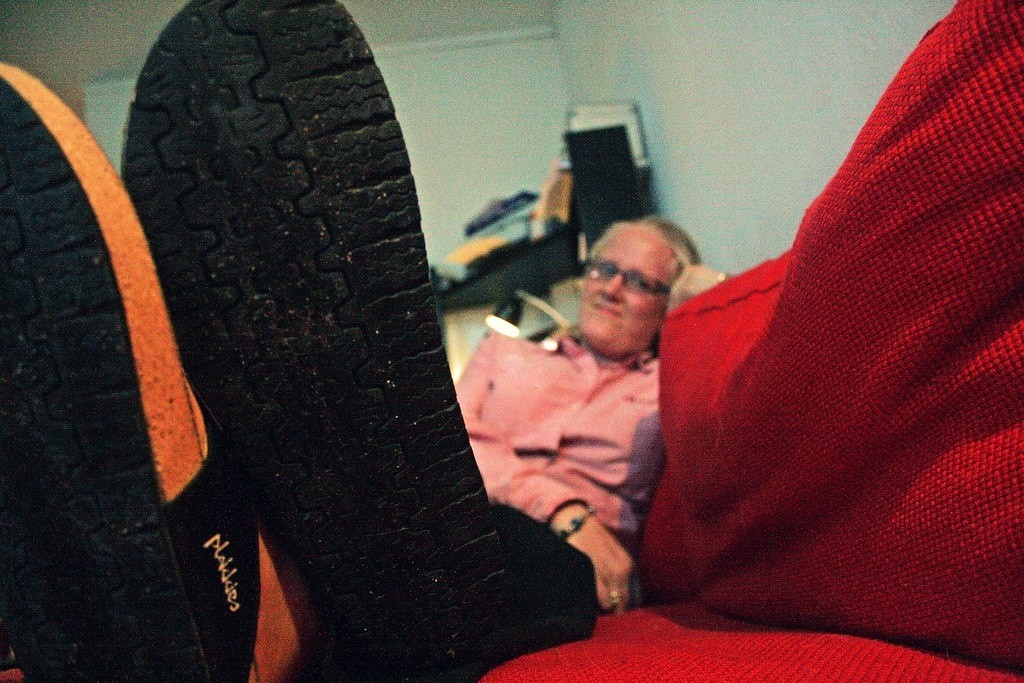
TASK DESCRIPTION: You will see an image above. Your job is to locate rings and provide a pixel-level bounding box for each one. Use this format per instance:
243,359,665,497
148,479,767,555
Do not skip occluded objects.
610,592,620,604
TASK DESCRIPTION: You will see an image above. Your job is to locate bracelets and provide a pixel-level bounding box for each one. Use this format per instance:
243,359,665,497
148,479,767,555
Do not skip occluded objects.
559,506,597,541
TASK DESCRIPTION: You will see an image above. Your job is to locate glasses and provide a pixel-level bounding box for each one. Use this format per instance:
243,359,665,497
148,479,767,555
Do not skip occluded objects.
591,261,670,294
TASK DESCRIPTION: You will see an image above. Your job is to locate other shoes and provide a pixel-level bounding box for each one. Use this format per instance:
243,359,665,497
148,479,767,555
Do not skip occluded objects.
0,61,335,683
119,0,511,683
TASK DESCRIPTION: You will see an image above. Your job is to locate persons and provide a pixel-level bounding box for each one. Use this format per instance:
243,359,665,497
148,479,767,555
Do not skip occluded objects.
0,0,703,683
